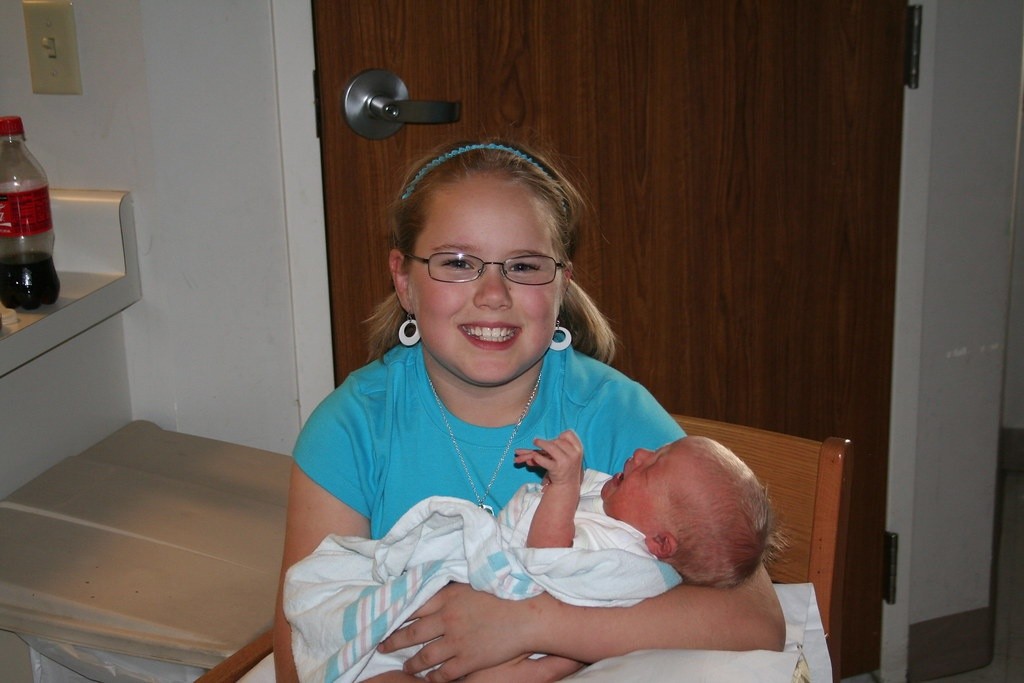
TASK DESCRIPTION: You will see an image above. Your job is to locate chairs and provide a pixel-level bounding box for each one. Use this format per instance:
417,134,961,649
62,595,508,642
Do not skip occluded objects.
193,413,856,683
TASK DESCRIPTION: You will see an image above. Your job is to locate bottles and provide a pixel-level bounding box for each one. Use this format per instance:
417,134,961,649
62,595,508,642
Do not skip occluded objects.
0,116,60,311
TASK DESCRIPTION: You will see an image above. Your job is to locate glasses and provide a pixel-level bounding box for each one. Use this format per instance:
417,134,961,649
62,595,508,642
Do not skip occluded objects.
395,244,564,286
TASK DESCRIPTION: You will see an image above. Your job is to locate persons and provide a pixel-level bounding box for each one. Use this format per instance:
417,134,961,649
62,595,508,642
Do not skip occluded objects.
272,132,787,683
286,429,771,683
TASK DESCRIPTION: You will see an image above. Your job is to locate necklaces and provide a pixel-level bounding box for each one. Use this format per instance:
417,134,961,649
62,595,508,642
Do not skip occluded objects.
424,368,543,517
